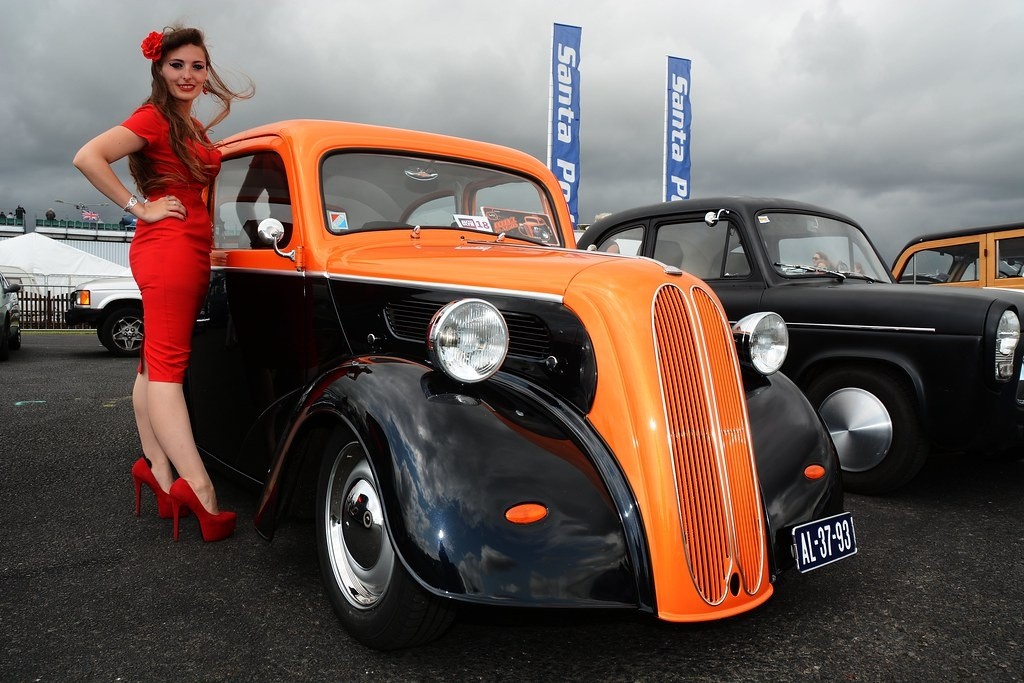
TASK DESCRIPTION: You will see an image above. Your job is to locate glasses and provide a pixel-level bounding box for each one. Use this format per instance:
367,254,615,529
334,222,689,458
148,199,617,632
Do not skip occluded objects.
812,257,821,261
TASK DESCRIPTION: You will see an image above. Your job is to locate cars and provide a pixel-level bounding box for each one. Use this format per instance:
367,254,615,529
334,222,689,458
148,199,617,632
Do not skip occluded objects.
0,272,22,358
574,198,1024,496
182,120,857,654
890,222,1024,291
65,277,144,358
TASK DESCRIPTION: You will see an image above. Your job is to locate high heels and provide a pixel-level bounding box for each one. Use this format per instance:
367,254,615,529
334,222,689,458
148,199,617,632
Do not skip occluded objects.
169,478,237,542
132,457,188,517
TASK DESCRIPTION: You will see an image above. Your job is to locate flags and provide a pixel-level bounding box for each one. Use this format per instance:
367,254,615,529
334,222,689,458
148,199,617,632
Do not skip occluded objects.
547,21,583,231
81,209,100,221
663,55,692,202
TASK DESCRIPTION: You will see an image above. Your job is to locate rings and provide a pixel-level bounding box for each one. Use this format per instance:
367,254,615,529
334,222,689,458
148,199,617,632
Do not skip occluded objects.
167,195,170,198
174,201,176,206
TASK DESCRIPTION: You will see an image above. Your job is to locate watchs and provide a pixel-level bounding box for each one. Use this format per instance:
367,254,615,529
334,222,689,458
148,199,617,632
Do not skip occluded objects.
123,194,138,212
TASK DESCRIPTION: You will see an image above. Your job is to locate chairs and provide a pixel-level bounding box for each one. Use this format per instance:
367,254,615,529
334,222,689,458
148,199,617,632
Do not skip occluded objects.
637,240,683,268
710,252,749,277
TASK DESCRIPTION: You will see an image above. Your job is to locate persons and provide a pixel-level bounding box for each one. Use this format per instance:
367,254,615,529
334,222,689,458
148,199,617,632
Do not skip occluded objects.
236,152,293,249
813,252,863,273
0,205,26,218
594,213,620,254
46,208,55,220
119,217,131,225
73,27,238,542
